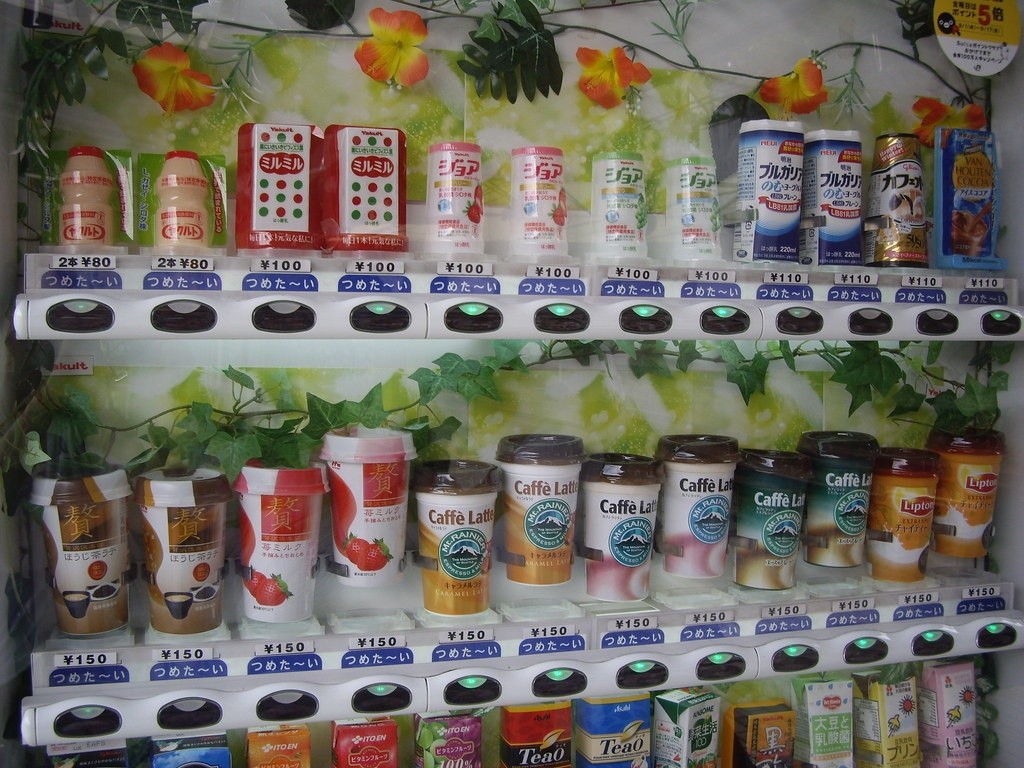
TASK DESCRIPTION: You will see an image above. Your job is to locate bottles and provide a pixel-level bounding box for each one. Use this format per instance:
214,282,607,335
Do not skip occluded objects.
61,147,111,248
155,151,212,253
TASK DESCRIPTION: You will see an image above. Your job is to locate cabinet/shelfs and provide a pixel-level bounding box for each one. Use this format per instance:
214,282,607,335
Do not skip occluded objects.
12,247,1023,746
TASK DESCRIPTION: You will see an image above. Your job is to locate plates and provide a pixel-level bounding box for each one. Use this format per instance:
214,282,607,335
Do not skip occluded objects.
89,581,123,601
193,584,219,600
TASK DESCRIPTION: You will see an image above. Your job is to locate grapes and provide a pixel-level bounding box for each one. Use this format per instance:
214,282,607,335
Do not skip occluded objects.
424,750,434,768
429,739,447,763
416,728,433,749
428,723,445,740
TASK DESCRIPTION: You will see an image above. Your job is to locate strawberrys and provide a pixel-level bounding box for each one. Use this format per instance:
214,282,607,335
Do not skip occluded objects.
256,575,292,605
358,539,393,570
342,534,369,564
249,572,267,597
559,188,566,205
475,183,482,205
548,204,564,226
463,201,480,223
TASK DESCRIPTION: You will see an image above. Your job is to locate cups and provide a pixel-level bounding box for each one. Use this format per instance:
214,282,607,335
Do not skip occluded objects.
580,453,657,602
136,466,229,634
871,449,936,581
797,429,877,569
736,448,805,591
60,592,92,619
29,458,130,634
928,426,1003,556
164,591,192,620
655,434,737,579
417,457,493,617
498,434,580,586
326,431,416,589
233,459,324,620
